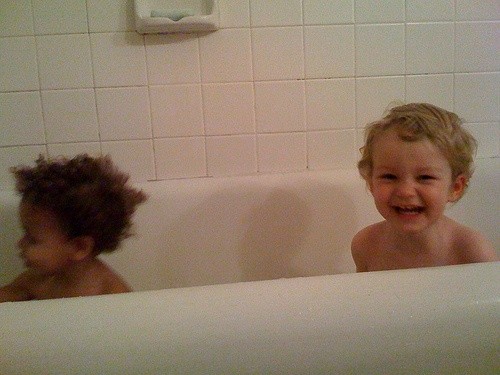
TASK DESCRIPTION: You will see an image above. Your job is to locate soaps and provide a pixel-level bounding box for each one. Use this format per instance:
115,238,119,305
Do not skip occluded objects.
150,7,195,21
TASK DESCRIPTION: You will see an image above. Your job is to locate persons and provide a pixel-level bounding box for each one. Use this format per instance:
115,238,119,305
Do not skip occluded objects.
351,101,494,272
0,153,150,303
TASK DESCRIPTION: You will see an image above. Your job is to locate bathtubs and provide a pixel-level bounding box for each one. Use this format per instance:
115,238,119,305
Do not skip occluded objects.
1,158,500,375
